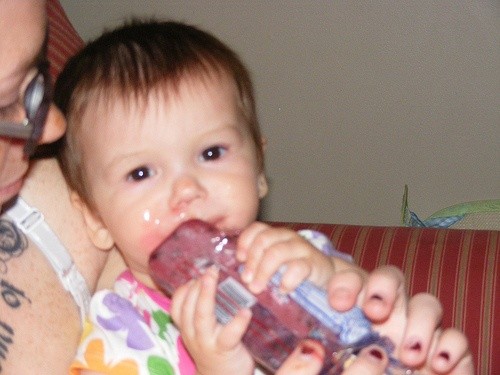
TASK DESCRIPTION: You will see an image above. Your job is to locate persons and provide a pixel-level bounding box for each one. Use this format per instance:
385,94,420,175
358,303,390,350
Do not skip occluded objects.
53,14,374,375
0,0,474,375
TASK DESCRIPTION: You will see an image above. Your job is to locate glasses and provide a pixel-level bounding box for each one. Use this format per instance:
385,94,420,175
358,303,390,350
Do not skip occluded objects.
0,60,51,162
